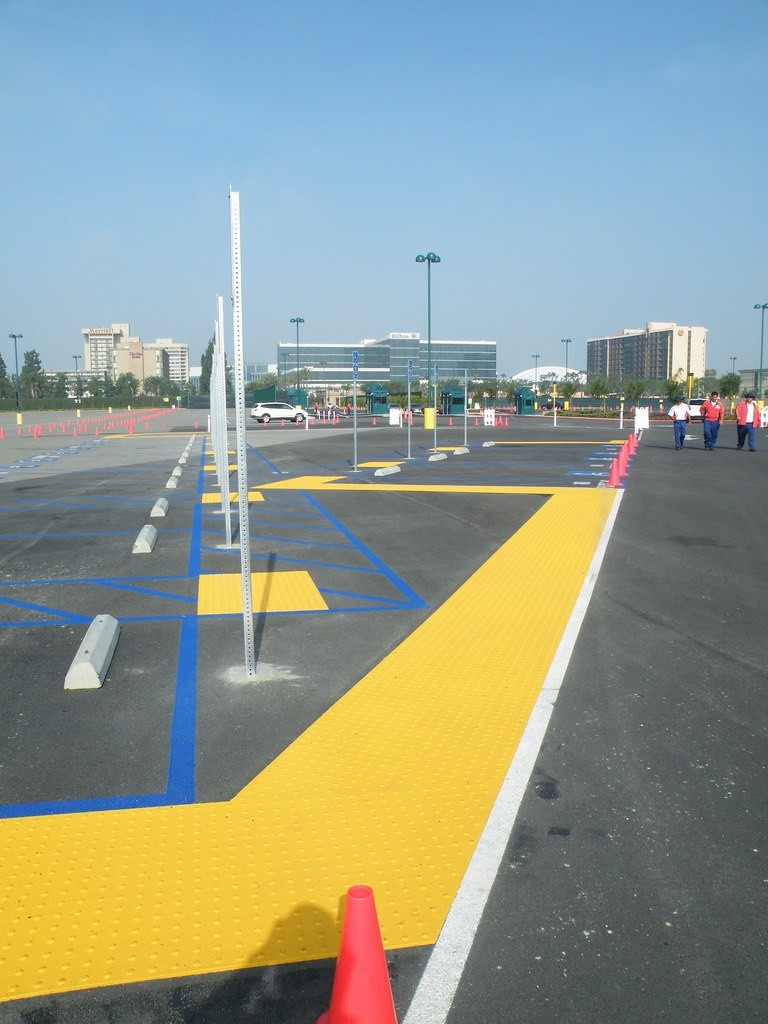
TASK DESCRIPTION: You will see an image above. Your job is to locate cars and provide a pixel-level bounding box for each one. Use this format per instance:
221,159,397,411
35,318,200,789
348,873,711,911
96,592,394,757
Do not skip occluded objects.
684,397,710,422
541,401,564,409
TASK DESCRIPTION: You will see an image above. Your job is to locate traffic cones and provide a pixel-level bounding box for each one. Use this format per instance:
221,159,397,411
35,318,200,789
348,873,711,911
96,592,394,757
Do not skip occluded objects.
312,883,398,1024
473,417,479,426
448,416,453,426
605,458,623,487
627,432,640,455
294,416,340,428
503,417,509,427
342,408,346,415
263,418,268,427
619,444,632,470
617,450,630,478
371,417,377,425
623,440,631,461
403,409,408,419
193,420,199,430
496,415,504,426
0,407,181,439
487,403,664,414
410,413,415,426
281,419,285,427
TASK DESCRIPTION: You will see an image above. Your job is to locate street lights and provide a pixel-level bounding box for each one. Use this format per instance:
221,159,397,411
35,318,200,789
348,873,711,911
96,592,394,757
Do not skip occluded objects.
729,356,739,394
289,317,306,405
72,354,82,409
7,333,24,408
281,352,290,403
414,253,441,408
561,338,571,382
752,302,768,401
531,354,540,397
275,366,282,402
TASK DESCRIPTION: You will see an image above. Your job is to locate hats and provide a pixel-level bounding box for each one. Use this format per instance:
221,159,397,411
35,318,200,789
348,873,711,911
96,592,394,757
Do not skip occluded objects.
674,396,681,401
744,392,756,398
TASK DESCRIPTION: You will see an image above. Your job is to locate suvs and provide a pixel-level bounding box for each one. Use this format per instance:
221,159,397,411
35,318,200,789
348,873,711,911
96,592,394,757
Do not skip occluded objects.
249,401,308,424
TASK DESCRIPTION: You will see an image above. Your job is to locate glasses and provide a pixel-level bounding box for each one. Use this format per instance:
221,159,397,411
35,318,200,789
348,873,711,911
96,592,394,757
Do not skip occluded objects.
747,396,752,398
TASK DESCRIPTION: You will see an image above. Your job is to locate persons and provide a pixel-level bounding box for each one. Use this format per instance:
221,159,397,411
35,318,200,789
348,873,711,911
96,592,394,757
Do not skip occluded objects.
314,401,318,413
736,392,760,451
700,391,724,450
347,402,352,414
668,395,690,450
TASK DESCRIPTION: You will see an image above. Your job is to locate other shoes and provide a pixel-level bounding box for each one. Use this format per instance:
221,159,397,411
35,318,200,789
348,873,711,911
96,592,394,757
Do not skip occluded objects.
750,449,756,452
681,444,684,449
705,446,709,450
675,447,679,450
737,445,743,450
711,448,716,451
